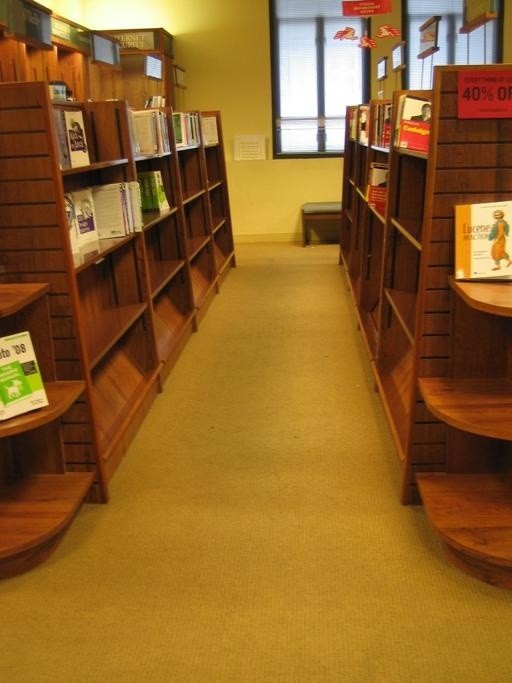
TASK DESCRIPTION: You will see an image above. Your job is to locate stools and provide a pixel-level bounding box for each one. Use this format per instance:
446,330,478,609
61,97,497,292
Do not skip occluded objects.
301,201,343,246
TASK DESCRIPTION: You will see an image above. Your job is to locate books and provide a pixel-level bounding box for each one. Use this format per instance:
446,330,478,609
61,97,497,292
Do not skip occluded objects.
51,106,219,269
348,93,431,208
1,330,51,421
453,199,512,282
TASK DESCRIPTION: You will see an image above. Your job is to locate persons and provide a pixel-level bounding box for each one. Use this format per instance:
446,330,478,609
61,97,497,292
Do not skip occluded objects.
487,209,512,272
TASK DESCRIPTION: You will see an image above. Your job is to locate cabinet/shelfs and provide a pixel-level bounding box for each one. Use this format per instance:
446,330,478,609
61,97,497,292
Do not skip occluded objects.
339,63,512,590
0,0,237,580
